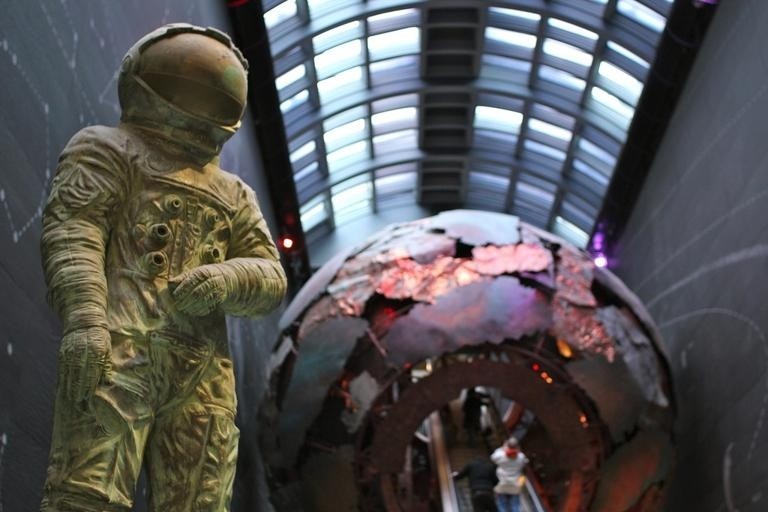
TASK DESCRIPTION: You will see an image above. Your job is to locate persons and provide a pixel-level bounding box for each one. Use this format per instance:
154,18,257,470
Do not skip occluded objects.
452,454,500,511
460,386,492,447
438,402,457,432
40,20,289,512
490,435,530,511
